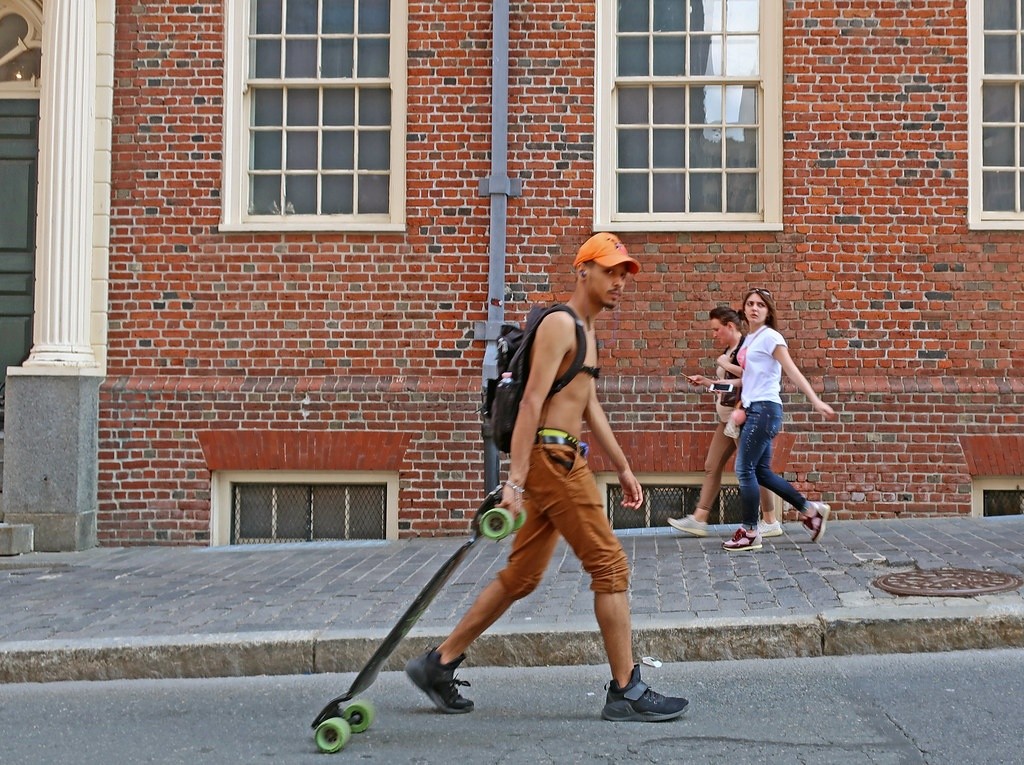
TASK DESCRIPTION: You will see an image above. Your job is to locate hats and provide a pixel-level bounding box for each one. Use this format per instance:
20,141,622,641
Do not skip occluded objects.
571,229,642,274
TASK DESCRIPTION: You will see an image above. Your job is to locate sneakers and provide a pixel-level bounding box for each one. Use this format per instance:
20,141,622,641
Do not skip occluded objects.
404,645,476,715
757,519,783,537
666,513,707,537
803,500,831,542
721,528,764,551
598,663,691,721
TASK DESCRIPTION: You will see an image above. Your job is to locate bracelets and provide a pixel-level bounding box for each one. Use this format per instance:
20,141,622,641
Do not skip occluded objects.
505,479,526,495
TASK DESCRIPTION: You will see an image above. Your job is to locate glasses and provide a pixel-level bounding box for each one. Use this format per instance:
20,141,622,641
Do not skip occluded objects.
749,287,772,297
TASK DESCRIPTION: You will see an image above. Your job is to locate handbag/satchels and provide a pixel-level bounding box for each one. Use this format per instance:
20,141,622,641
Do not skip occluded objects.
720,335,743,408
722,400,746,439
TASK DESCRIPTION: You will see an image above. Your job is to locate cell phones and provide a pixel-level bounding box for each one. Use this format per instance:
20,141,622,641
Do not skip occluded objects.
710,383,733,393
680,373,698,386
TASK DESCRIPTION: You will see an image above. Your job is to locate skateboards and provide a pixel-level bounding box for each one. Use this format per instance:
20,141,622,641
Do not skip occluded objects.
311,486,527,753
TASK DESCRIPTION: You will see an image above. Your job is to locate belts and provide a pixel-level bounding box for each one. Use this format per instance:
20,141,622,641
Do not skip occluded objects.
533,433,587,456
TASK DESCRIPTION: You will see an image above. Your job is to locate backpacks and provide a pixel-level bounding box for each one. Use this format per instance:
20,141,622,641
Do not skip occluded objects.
478,301,589,453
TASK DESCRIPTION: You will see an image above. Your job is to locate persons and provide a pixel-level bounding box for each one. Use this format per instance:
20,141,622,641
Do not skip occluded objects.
665,305,786,537
402,230,695,723
714,287,841,551
